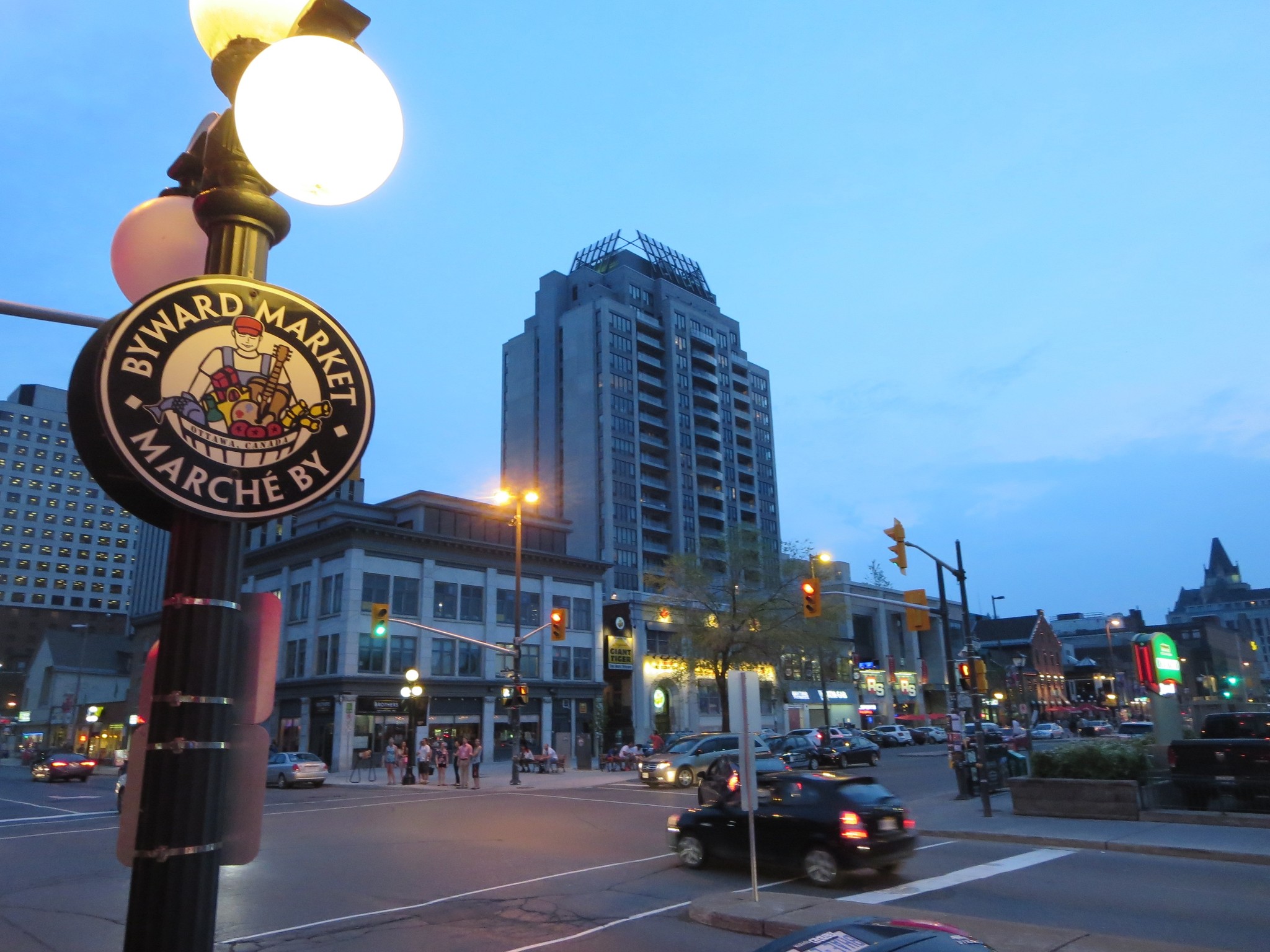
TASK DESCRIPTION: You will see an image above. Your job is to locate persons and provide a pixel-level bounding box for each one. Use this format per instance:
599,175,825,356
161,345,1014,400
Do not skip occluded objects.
517,743,534,773
650,729,666,754
453,738,483,789
535,743,559,774
382,737,409,785
435,741,448,786
606,742,646,773
416,738,433,784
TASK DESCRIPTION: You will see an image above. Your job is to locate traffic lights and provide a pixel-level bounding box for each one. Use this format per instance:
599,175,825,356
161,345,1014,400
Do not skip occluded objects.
1223,691,1230,700
883,518,908,569
1226,675,1239,689
802,577,822,618
371,603,388,638
516,685,528,696
958,662,970,679
551,608,565,641
501,685,514,698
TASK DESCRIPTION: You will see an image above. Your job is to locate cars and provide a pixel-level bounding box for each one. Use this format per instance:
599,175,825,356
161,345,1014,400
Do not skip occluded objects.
1116,720,1154,739
1078,720,1114,737
638,731,772,789
265,751,329,789
1028,723,1068,740
753,915,999,952
17,742,98,784
759,720,1028,771
665,768,920,890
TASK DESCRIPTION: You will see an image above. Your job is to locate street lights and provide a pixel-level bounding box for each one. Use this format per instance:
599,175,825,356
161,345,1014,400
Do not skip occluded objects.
400,669,423,785
493,490,540,786
991,594,1013,720
122,0,406,951
1105,619,1120,706
809,552,830,725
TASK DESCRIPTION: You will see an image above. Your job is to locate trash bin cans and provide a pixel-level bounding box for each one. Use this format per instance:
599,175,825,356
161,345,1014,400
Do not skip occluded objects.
946,731,1029,799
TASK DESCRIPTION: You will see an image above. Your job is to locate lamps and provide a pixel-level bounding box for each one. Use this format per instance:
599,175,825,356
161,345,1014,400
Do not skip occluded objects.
612,591,617,597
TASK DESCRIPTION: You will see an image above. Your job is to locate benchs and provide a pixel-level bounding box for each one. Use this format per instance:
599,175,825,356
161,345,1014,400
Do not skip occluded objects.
598,753,635,772
528,753,566,773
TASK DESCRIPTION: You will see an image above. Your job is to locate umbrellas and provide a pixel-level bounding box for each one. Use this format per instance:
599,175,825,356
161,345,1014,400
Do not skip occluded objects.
1045,704,1109,722
894,713,947,729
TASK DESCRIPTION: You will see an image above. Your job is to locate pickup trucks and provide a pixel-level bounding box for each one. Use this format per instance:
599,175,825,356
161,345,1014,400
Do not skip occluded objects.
1166,710,1270,815
